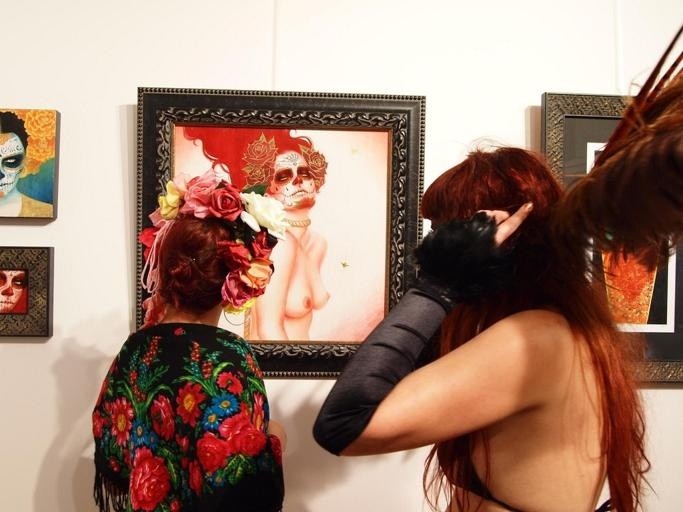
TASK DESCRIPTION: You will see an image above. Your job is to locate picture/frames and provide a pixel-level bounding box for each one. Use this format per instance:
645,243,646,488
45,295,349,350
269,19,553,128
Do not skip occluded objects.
542,92,683,389
137,87,426,380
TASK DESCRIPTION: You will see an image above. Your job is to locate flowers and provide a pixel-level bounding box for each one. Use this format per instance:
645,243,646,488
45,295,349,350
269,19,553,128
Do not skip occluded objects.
137,169,279,332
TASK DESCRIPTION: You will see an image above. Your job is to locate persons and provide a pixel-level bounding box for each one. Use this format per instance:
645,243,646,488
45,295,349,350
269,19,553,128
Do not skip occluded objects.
182,126,332,340
594,236,667,324
92,215,284,512
0,268,29,314
0,111,54,218
311,141,657,511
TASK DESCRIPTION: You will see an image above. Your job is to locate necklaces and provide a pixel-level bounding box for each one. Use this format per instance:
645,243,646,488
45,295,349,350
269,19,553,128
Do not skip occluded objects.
281,217,311,229
